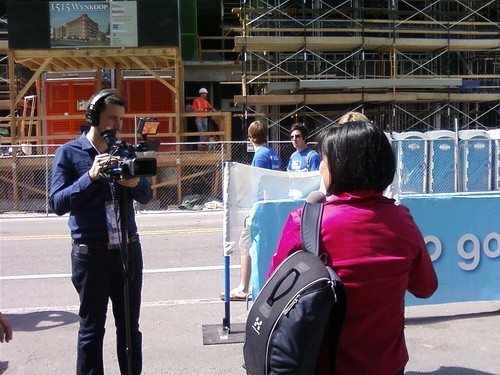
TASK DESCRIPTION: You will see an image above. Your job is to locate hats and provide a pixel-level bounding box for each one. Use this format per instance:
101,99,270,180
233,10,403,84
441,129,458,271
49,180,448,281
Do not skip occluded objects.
199,88,208,94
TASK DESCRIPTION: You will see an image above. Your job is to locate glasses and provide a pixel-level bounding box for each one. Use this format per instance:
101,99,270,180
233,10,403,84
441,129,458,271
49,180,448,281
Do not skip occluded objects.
290,135,300,139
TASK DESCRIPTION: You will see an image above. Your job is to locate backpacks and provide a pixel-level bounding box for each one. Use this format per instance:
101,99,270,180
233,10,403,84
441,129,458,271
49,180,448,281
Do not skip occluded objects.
242,201,348,375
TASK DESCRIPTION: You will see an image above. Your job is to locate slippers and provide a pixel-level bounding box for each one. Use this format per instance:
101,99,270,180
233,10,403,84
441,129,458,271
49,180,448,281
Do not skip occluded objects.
219,287,253,301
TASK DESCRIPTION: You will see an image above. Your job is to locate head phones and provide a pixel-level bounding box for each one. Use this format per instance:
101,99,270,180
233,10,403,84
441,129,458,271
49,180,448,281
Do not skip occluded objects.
85,91,113,126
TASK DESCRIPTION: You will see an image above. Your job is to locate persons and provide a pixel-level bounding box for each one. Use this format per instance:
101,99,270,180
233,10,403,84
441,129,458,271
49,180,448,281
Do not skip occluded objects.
286,123,323,200
219,120,282,301
192,88,217,149
0,312,12,343
48,90,153,375
268,120,439,375
